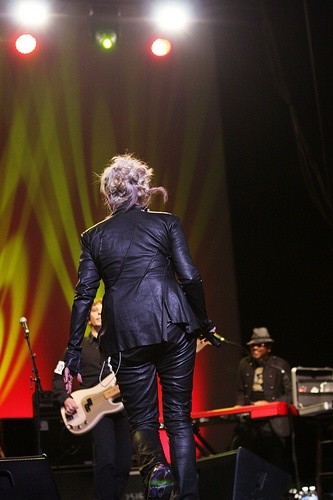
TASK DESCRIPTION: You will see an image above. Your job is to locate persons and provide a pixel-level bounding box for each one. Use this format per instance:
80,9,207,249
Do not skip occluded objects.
63,155,216,500
54,300,133,500
233,327,291,500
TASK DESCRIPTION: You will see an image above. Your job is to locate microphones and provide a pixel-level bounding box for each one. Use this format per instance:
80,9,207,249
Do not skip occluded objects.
20,316,30,338
205,332,221,348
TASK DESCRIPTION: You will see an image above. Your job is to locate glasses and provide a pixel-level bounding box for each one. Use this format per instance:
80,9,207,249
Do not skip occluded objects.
252,342,270,348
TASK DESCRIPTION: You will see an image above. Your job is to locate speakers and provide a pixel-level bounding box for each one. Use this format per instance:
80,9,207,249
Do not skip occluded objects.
0,454,60,500
0,418,37,458
194,447,291,500
36,416,95,473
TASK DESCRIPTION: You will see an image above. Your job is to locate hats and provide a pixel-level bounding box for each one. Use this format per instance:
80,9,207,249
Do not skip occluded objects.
247,327,274,346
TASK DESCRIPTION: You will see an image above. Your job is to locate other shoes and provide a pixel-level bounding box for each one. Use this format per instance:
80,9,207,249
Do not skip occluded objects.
145,461,174,500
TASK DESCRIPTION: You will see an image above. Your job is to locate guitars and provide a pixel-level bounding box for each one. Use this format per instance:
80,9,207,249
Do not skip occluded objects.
60,335,213,435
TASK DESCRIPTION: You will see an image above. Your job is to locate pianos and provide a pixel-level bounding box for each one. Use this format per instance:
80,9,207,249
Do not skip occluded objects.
158,401,298,500
